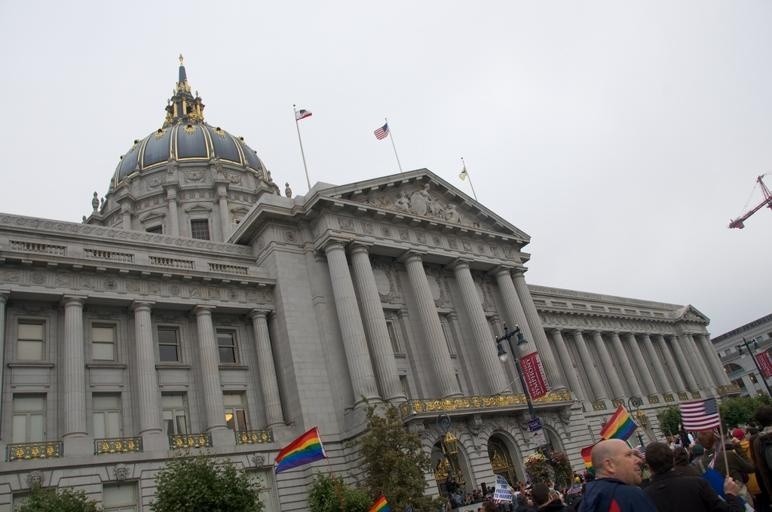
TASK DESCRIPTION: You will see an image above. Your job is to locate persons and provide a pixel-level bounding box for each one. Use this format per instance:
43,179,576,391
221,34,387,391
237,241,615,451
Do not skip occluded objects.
631,422,772,511
578,438,659,511
753,406,771,512
446,474,583,511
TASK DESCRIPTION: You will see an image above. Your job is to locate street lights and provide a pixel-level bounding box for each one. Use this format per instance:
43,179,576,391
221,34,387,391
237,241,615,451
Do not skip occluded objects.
735,336,772,397
496,323,551,459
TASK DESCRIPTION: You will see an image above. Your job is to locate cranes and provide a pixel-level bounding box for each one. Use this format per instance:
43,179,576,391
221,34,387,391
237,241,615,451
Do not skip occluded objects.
728,174,771,229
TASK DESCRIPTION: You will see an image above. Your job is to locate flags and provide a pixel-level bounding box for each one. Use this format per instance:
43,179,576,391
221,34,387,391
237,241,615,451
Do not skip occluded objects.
519,351,551,401
580,405,638,478
677,396,720,430
366,494,391,512
374,123,390,141
274,426,328,476
296,109,312,120
755,349,772,379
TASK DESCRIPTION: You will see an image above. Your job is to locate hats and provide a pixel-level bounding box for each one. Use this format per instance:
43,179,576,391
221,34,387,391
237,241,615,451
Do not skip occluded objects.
733,430,744,439
